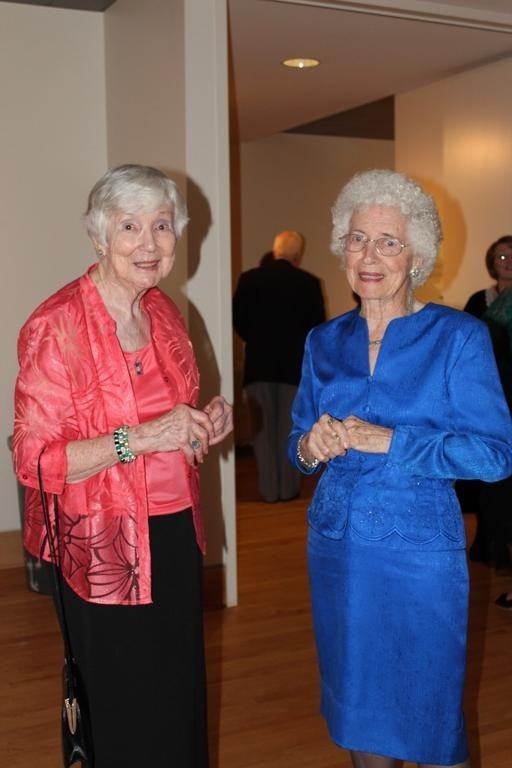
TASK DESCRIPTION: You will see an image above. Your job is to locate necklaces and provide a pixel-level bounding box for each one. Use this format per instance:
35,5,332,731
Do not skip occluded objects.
369,295,415,345
99,283,143,374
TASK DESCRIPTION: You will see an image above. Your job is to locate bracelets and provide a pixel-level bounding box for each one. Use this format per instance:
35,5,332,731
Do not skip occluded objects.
113,424,136,463
296,434,318,469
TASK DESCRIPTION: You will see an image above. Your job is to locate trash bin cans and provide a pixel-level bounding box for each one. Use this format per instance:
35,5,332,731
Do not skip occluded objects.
7,434,56,596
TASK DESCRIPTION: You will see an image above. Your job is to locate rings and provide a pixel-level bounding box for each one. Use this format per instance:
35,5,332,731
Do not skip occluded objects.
327,418,336,425
192,439,200,449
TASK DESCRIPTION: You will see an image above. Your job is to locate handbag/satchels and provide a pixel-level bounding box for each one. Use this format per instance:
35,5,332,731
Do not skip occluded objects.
59,660,89,768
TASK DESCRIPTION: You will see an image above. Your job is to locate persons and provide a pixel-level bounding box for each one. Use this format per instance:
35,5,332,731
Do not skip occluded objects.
233,231,327,503
11,164,233,768
287,170,512,768
464,235,512,609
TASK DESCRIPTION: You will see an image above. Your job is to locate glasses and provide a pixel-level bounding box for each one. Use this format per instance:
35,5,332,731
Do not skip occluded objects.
338,233,410,257
